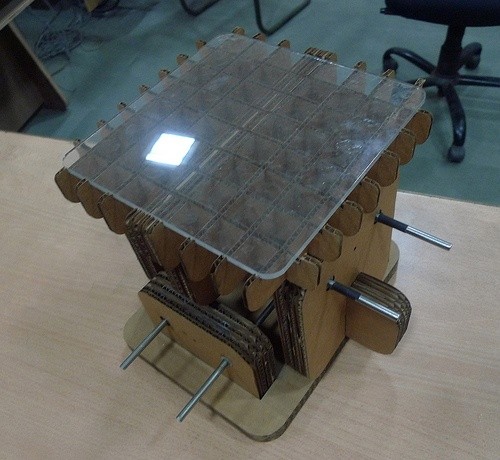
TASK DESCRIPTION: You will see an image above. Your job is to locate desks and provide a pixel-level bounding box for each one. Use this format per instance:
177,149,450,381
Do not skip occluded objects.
0,131,500,460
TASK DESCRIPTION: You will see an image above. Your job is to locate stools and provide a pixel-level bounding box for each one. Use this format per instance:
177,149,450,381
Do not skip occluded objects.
380,0,500,164
